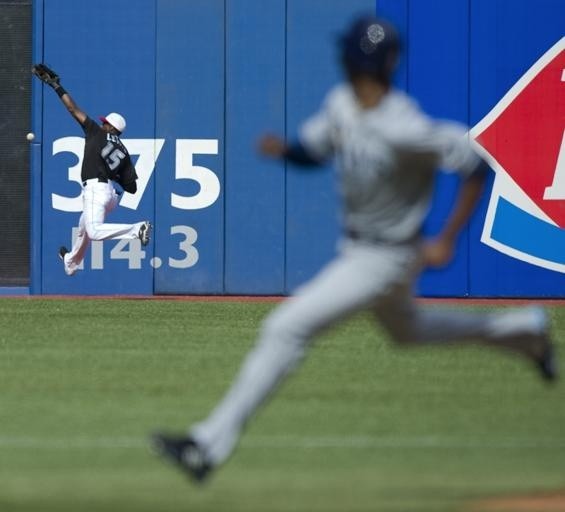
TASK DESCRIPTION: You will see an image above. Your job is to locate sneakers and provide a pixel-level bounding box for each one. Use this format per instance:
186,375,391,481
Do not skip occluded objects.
537,345,554,381
150,433,211,482
58,246,69,260
139,220,152,245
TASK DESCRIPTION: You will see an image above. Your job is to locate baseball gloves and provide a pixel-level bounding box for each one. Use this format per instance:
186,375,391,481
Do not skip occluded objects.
32,64,59,87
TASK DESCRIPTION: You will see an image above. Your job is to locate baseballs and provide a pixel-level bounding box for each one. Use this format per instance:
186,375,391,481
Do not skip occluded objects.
26,133,34,141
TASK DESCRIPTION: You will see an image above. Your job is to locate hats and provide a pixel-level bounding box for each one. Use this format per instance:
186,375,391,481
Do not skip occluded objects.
99,112,126,133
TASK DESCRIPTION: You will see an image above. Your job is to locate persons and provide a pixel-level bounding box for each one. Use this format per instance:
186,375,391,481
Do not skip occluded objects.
156,14,557,490
33,63,150,277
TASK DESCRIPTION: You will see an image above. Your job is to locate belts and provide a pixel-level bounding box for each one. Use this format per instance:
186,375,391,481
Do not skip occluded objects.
83,177,108,186
